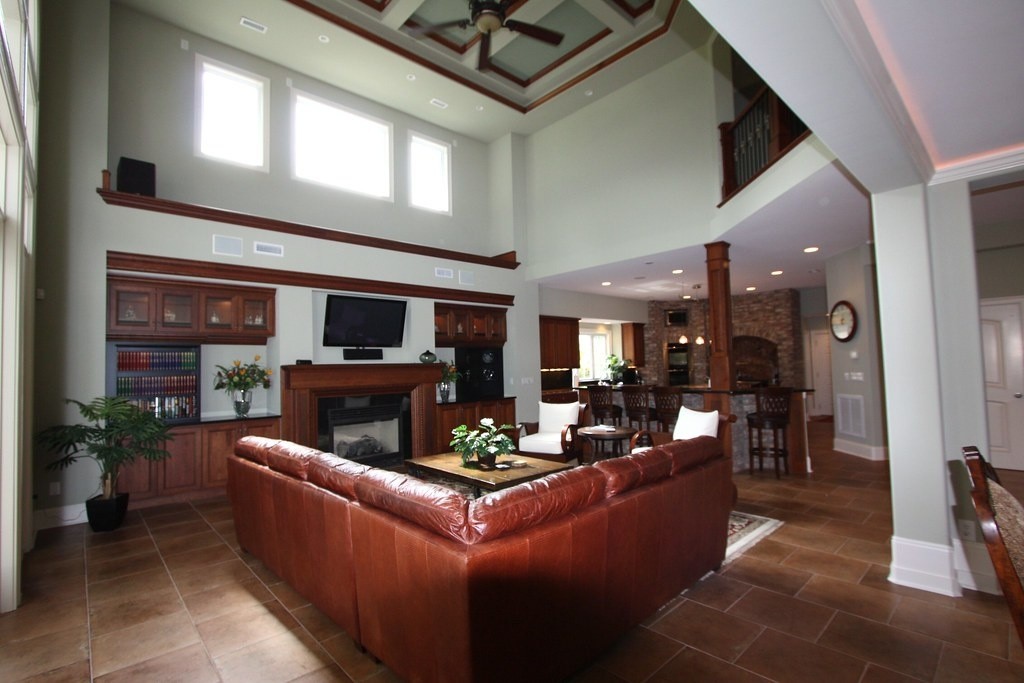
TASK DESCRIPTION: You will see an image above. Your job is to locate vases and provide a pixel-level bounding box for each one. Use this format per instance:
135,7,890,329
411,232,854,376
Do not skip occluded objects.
231,390,252,418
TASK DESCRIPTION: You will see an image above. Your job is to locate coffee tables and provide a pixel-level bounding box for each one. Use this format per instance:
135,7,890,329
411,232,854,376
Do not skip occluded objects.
403,451,574,499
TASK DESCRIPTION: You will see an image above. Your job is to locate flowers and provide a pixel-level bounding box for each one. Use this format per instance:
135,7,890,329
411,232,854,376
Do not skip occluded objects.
214,354,272,393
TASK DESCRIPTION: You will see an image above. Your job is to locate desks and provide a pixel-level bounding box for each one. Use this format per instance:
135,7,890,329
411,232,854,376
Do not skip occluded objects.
577,427,637,465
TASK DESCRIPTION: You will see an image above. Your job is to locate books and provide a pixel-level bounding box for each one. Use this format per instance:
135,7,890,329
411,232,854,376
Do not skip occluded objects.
117,351,196,422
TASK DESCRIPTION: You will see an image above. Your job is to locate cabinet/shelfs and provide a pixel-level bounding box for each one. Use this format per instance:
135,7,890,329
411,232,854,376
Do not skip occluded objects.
203,418,282,498
434,302,471,345
433,403,476,453
199,283,276,337
106,340,201,424
470,305,508,342
539,315,581,368
111,424,202,500
106,276,199,339
477,397,516,456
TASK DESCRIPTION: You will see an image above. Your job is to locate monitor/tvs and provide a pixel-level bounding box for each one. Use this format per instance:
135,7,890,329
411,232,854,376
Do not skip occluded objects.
323,294,407,348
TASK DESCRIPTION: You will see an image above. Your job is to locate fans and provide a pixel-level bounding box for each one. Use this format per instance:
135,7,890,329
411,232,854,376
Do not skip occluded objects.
415,0,562,71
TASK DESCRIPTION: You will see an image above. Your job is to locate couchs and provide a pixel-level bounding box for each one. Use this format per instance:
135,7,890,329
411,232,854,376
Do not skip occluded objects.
226,434,736,683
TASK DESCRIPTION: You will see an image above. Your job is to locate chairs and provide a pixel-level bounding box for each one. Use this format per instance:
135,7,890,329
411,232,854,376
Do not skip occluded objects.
961,445,1024,647
631,410,737,476
519,404,591,463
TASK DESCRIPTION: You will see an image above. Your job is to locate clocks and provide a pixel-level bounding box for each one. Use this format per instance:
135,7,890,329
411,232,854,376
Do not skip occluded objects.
830,300,857,343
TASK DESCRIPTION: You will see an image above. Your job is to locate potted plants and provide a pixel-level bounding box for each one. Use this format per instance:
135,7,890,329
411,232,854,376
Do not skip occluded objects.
36,397,177,532
450,418,516,466
606,353,633,385
437,359,462,403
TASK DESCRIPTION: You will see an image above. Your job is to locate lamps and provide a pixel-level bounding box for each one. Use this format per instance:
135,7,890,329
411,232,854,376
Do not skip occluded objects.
695,335,704,346
679,334,688,344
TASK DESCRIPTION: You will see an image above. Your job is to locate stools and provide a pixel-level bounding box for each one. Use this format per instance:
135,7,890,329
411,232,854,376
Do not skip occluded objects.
747,386,793,480
587,385,625,457
621,386,654,449
653,386,682,432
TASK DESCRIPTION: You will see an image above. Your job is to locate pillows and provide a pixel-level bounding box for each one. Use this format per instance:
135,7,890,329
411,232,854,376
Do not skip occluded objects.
539,402,579,438
672,404,719,440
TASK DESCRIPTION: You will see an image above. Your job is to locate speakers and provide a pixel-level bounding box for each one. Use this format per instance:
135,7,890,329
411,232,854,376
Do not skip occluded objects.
116,157,156,198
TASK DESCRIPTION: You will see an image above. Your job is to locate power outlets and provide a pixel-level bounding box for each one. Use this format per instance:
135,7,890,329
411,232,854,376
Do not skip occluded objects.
49,482,60,495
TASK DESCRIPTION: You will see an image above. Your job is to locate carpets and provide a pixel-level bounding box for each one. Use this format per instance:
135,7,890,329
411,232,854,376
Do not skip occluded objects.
657,512,786,614
